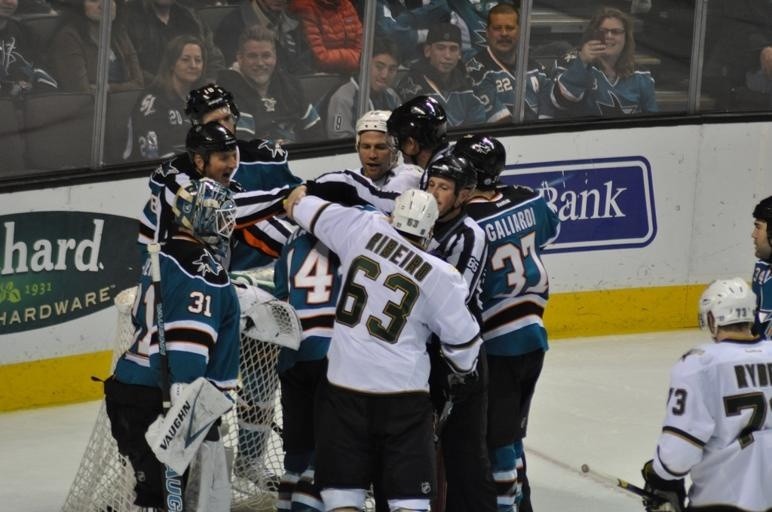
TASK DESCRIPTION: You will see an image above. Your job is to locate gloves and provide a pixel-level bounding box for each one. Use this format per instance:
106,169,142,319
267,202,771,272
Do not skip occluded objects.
642,460,686,512
441,359,478,404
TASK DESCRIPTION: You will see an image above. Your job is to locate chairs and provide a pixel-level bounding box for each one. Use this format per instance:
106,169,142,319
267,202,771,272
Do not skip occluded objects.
1,2,570,177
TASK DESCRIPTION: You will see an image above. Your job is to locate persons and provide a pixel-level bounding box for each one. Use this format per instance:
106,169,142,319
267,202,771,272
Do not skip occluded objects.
749,196,772,338
705,0,772,110
551,6,660,119
639,277,772,512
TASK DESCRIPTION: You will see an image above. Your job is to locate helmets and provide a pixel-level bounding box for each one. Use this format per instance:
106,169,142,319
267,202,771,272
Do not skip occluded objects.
171,82,239,252
354,96,505,240
699,277,757,339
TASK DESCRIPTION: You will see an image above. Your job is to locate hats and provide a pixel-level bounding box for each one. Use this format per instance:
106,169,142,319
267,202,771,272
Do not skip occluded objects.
426,23,461,44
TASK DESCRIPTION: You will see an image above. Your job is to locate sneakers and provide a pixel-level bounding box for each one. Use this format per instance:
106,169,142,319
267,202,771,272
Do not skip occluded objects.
234,454,279,490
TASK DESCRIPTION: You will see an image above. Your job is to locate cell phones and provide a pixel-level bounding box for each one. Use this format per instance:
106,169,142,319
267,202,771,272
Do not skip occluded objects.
593,28,605,43
